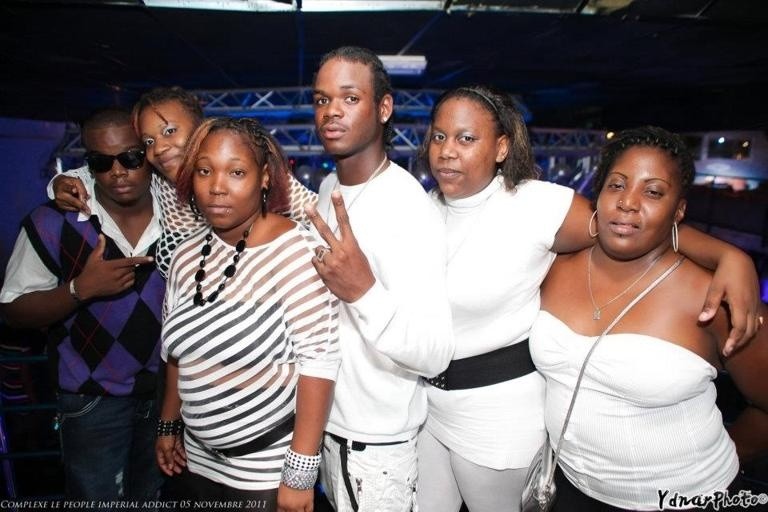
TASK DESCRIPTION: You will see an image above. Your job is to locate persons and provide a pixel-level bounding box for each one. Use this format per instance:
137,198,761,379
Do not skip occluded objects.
47,88,320,511
528,127,768,509
1,103,186,511
306,45,456,512
417,83,765,511
155,115,342,511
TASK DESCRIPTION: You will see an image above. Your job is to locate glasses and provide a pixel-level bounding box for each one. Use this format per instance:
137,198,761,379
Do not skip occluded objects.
86,150,146,173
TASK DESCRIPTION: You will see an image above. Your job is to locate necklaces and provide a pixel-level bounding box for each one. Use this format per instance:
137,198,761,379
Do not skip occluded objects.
194,225,252,306
316,151,390,260
588,241,661,322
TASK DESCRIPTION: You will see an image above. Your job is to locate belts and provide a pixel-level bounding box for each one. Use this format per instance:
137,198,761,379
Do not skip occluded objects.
422,338,536,391
185,415,295,463
329,434,409,451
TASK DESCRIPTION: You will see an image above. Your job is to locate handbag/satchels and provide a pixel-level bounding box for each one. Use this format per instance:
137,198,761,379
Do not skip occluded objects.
520,441,557,512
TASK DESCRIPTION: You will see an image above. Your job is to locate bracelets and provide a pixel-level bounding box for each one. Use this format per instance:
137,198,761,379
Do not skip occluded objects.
70,278,86,304
154,418,182,438
281,465,318,489
285,448,322,472
